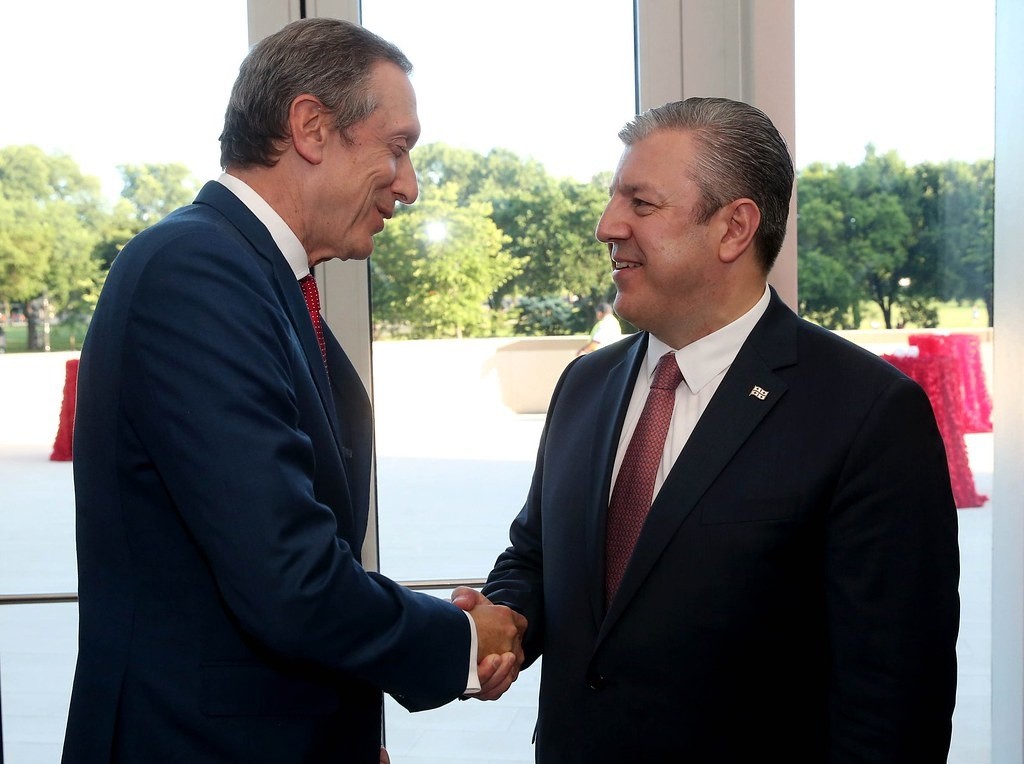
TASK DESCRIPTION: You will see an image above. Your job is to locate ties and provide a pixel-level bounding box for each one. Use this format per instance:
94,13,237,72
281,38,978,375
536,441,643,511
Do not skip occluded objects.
607,347,685,611
295,263,334,424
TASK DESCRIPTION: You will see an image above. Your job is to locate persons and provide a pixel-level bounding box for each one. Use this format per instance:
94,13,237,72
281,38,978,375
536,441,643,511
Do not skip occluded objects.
452,98,961,764
60,16,529,763
